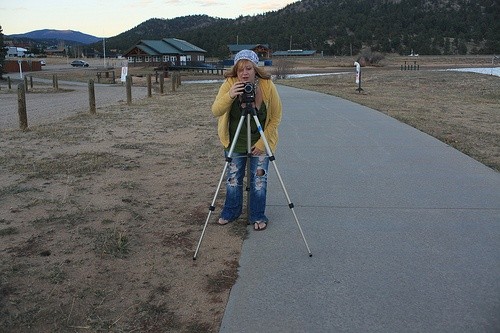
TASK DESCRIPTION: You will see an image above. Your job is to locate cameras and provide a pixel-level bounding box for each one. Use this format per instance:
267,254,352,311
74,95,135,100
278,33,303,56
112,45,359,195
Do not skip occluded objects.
237,82,256,104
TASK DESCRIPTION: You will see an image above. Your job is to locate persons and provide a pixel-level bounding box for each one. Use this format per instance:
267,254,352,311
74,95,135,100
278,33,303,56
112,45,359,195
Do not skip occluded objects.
212,50,282,231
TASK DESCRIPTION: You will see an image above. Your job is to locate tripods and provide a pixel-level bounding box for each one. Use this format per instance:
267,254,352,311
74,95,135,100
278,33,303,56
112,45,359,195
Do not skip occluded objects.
192,95,313,259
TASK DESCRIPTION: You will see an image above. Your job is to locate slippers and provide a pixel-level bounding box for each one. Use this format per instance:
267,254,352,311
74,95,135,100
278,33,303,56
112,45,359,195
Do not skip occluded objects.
218,216,229,225
254,221,267,231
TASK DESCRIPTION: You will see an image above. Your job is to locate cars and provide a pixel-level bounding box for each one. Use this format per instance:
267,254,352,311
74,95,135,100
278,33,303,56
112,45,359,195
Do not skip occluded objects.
39,59,46,65
71,60,89,67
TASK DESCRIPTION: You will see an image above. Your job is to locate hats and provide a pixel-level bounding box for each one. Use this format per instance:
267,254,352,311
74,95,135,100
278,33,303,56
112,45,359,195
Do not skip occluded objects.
233,49,259,67
